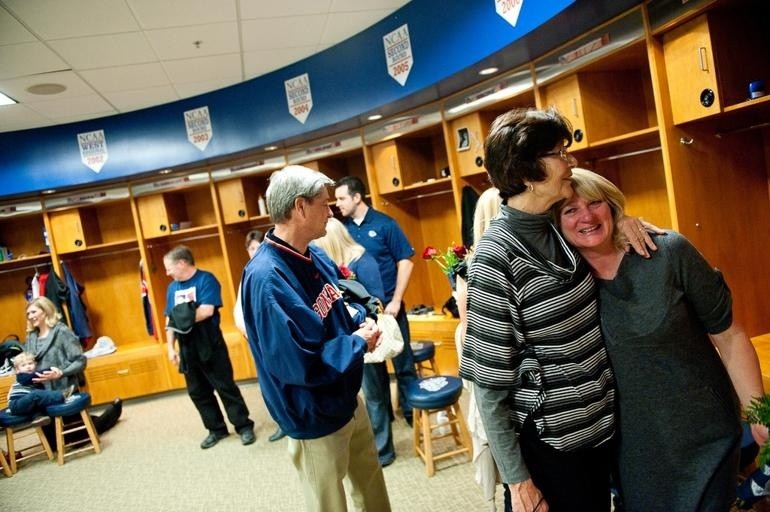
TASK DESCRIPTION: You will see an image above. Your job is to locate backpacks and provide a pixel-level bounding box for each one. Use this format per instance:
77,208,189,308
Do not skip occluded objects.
0,332,22,370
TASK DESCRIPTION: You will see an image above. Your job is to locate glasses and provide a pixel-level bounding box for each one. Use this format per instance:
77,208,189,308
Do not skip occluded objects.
539,146,569,162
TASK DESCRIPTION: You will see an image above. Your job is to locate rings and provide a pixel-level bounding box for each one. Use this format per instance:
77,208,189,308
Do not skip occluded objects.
638,227,645,232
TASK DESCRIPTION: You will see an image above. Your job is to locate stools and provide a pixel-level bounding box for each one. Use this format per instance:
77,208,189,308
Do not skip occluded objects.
0,390,101,476
395,339,474,477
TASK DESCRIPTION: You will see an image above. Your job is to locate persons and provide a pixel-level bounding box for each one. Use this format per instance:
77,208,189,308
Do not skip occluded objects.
7,353,76,415
455,187,503,511
555,168,770,512
459,106,669,512
163,245,255,449
23,296,123,451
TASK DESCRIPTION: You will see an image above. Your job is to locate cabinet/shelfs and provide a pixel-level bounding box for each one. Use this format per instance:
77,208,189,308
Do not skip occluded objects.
295,67,531,377
2,150,284,407
534,3,770,394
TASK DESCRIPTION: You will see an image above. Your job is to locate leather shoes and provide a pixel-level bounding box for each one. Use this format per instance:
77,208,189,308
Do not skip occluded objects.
240,428,256,446
198,428,230,449
269,426,287,442
